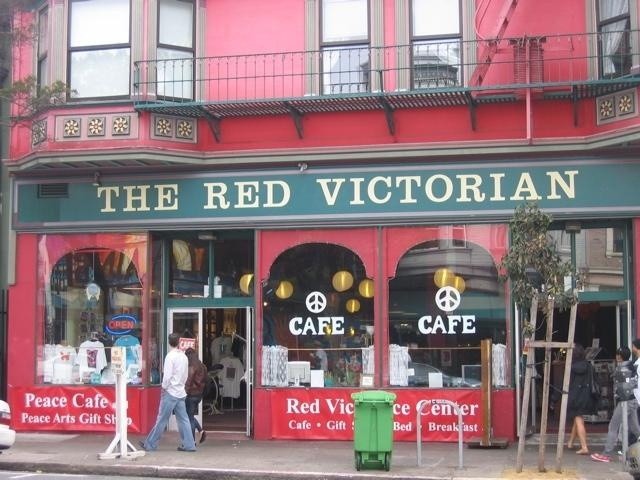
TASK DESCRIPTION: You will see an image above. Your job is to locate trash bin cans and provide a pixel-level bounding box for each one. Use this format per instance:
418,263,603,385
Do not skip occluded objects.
351,390,397,472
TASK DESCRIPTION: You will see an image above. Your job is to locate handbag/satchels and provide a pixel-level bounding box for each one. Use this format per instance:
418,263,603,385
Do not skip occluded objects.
589,373,600,397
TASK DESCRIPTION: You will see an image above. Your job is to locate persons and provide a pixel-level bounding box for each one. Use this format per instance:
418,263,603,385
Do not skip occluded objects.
310,340,328,371
560,339,640,463
137,333,209,452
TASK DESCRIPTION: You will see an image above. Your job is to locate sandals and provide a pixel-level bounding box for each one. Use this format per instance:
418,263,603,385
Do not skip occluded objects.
568,443,575,449
575,450,589,455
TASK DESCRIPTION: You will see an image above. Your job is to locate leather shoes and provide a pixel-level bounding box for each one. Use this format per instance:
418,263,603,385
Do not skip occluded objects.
177,447,199,451
138,441,145,449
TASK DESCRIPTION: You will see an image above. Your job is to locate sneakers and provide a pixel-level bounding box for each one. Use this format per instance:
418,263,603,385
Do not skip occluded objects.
200,431,206,444
590,452,609,463
